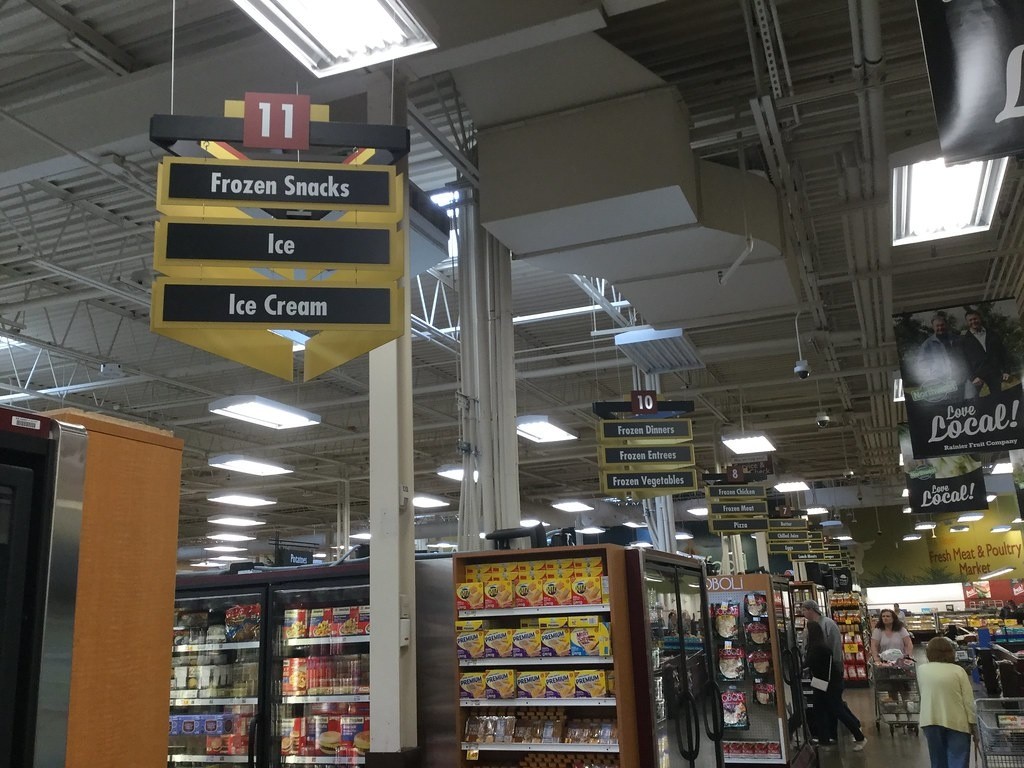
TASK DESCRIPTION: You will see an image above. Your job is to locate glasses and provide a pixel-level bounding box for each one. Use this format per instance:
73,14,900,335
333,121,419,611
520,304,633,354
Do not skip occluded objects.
1006,602,1010,605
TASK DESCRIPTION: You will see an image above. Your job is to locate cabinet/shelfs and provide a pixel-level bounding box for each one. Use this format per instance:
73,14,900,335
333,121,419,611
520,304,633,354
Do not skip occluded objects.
452,544,640,768
935,611,999,637
827,591,874,687
792,581,832,645
871,614,937,642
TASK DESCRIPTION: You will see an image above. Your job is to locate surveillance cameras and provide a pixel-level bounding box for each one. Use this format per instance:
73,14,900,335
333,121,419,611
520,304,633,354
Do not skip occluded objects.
794,360,813,380
848,513,853,519
853,520,858,525
816,410,831,428
834,514,840,521
845,468,855,481
877,531,883,536
857,493,863,501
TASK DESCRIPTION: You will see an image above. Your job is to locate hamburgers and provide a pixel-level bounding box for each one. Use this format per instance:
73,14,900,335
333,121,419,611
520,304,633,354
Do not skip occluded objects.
354,730,370,754
318,731,341,755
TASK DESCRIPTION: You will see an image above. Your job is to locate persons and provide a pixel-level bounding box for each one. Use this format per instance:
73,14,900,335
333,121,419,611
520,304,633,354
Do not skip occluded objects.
870,609,914,731
796,601,867,751
1001,599,1024,623
916,637,978,768
895,605,903,623
917,309,1011,405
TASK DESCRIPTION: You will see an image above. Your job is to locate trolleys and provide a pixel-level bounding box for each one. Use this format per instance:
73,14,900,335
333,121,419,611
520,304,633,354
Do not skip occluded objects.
873,660,922,738
973,698,1024,768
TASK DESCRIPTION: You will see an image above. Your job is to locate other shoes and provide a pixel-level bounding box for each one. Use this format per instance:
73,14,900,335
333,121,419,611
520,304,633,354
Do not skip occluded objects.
812,739,834,744
853,738,868,752
853,727,863,743
895,719,902,728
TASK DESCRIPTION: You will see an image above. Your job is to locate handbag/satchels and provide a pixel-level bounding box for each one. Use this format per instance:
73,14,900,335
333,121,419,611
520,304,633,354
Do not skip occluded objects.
810,677,828,691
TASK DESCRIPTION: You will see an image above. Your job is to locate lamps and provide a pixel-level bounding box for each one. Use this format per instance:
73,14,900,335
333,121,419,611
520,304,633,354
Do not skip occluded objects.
899,453,1024,580
412,493,449,510
615,328,707,377
191,532,257,570
721,394,777,455
207,395,321,430
889,139,1009,247
205,492,279,508
552,499,593,513
235,0,440,79
208,454,295,477
893,378,906,402
520,516,551,528
624,505,709,547
437,463,480,482
207,514,266,528
573,525,606,534
774,478,811,493
803,503,852,541
515,415,579,443
